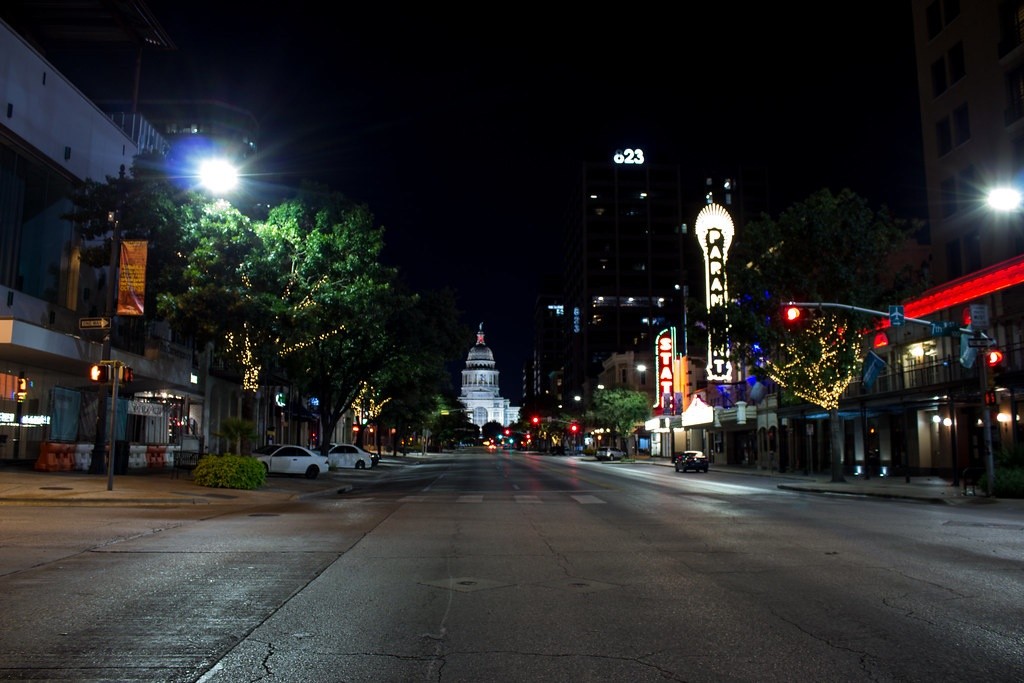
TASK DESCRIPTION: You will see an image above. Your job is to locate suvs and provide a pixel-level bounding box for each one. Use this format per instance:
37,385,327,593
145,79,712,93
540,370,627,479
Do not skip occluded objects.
595,446,628,462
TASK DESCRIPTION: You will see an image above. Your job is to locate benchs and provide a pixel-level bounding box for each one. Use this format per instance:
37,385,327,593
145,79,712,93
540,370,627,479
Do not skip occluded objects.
169,451,209,477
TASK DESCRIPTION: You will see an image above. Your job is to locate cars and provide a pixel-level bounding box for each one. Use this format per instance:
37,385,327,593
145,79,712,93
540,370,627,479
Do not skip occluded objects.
674,450,709,474
309,442,380,471
246,443,331,480
484,437,543,454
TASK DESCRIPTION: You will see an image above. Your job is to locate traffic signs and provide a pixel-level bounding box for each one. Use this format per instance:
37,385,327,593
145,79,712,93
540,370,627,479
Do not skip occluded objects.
967,338,998,349
79,316,112,330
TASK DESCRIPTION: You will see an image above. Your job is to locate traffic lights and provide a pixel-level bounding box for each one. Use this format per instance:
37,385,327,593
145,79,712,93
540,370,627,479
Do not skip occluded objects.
984,349,1004,369
985,390,999,408
570,423,578,433
120,365,133,384
90,363,110,383
532,416,539,425
784,303,802,333
504,427,510,436
